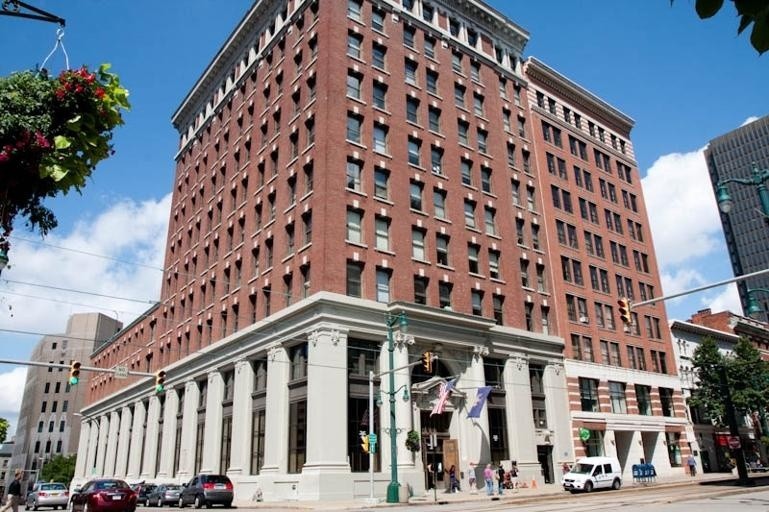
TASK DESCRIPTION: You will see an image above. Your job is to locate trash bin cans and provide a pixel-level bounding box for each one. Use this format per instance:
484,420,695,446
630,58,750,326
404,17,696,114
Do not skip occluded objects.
632,464,657,482
397,485,410,503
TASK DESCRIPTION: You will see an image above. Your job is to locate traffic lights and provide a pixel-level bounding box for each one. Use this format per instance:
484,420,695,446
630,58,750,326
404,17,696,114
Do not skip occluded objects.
419,351,434,375
69,360,83,386
616,294,634,327
155,371,167,396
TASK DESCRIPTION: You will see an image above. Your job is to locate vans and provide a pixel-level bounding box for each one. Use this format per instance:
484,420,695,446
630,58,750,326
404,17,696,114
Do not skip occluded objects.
559,455,622,493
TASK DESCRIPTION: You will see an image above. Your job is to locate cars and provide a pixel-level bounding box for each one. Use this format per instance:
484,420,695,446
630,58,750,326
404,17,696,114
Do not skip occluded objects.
69,478,138,511
24,482,70,511
128,480,184,507
178,473,234,508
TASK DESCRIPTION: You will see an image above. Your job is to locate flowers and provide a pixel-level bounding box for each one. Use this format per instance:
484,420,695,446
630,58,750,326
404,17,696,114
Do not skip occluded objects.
0,58,133,257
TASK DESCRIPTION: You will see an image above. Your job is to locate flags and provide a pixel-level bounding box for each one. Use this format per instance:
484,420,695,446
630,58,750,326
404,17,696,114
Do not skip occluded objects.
466,386,492,419
430,376,459,418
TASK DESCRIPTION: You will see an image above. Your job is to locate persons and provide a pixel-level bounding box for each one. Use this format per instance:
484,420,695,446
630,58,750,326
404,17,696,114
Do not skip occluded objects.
484,463,494,495
509,461,518,488
496,464,504,495
468,462,478,494
1,469,24,512
503,471,513,489
449,465,459,493
687,454,696,477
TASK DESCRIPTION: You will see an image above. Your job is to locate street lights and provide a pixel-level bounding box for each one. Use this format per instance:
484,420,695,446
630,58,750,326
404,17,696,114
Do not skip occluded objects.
373,307,417,503
716,157,768,322
